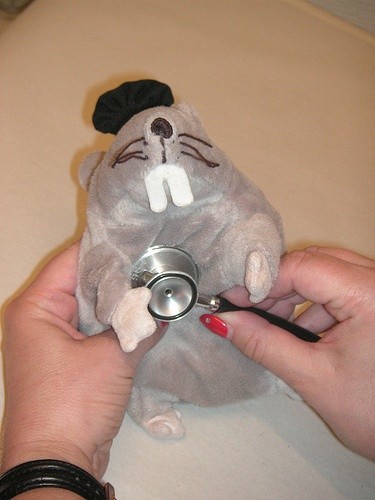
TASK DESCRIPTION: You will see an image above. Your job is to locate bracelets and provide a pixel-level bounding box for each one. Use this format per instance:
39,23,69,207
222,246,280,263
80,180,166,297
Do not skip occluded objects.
2,458,114,499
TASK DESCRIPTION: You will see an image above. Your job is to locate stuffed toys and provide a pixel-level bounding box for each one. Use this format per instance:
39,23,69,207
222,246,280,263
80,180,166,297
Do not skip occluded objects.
72,79,286,446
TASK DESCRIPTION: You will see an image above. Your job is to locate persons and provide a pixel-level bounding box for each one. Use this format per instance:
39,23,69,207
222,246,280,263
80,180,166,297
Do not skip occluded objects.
0,236,375,500
